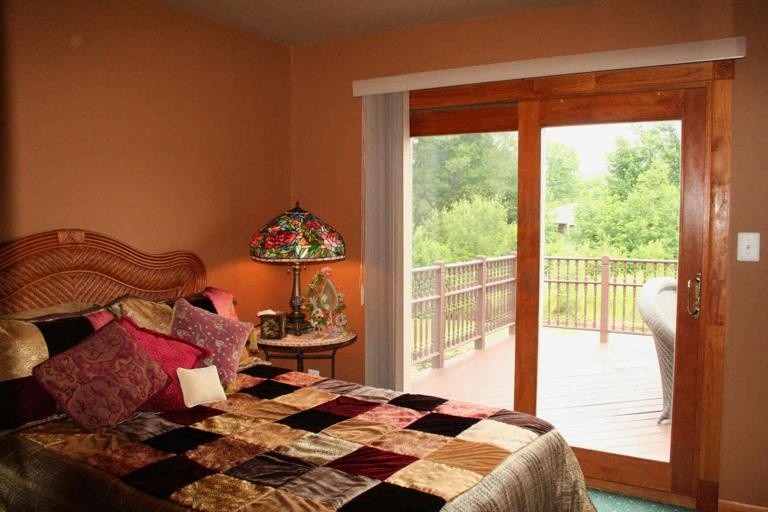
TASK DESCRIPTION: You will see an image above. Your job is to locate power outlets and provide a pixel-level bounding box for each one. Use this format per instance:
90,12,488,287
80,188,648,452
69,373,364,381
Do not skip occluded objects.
307,369,319,377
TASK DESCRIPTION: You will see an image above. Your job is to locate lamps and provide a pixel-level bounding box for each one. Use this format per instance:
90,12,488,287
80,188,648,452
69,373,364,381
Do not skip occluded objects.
249,201,346,335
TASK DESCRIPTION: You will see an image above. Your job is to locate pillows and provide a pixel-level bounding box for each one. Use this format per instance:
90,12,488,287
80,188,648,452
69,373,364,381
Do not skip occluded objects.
0,306,117,435
176,366,228,408
169,298,254,387
111,286,271,371
32,318,169,432
119,312,214,418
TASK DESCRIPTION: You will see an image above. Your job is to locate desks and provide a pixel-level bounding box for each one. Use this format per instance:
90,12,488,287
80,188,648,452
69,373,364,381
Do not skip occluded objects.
250,322,358,380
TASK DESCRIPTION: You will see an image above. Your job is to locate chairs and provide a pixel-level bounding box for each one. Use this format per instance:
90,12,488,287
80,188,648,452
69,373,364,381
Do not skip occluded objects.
636,275,676,424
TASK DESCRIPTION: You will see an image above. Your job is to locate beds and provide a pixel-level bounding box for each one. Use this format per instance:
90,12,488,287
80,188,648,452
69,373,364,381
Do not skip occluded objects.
0,227,597,512
